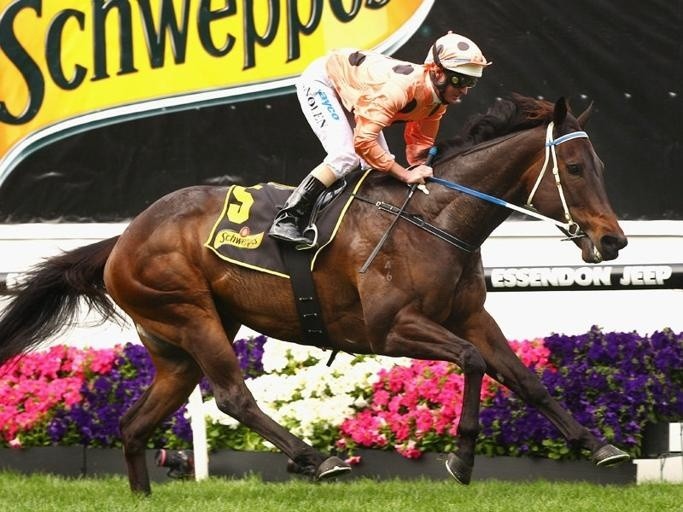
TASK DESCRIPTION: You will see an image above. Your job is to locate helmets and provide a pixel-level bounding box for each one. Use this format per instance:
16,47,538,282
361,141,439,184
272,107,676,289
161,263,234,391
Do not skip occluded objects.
425,31,493,78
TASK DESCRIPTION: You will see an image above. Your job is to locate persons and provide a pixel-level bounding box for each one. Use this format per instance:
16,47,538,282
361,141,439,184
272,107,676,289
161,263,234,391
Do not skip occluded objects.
269,30,494,244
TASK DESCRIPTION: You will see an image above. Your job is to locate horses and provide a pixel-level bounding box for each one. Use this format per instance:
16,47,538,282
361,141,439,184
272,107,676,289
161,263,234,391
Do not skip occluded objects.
0,88,632,498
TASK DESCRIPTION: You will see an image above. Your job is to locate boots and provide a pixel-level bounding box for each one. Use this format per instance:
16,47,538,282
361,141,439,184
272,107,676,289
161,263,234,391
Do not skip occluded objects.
269,175,326,241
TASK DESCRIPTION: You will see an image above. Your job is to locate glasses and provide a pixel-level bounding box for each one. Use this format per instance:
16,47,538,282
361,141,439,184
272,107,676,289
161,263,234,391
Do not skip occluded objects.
449,73,477,88
